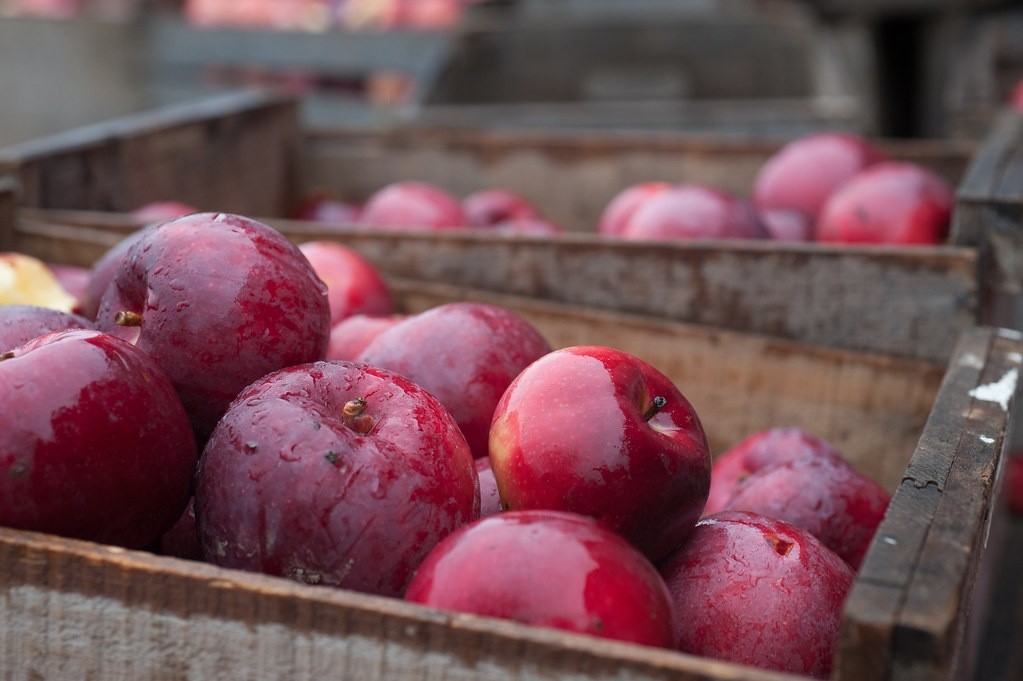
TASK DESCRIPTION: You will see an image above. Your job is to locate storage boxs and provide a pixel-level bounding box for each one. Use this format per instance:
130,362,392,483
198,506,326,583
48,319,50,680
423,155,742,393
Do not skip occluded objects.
0,80,1023,681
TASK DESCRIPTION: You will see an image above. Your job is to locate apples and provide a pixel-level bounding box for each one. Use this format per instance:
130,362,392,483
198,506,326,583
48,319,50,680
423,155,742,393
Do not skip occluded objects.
122,133,957,248
0,211,896,681
